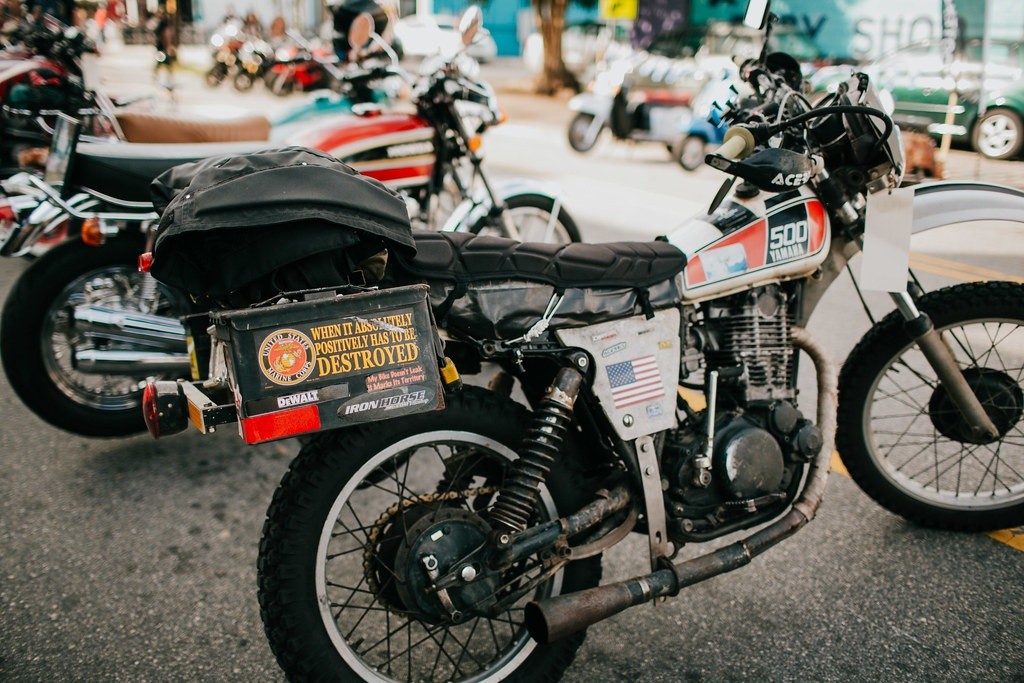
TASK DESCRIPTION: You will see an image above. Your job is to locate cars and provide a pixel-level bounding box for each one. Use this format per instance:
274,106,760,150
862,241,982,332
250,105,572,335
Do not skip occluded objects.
810,42,1023,163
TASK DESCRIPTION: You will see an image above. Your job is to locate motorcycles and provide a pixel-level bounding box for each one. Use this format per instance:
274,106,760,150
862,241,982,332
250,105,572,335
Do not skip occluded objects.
0,1,743,443
142,51,1024,683
